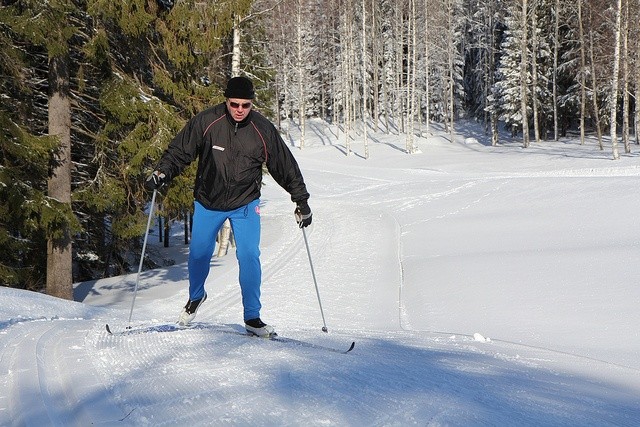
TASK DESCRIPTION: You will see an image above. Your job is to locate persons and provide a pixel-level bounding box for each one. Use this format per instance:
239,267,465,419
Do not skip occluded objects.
147,75,313,336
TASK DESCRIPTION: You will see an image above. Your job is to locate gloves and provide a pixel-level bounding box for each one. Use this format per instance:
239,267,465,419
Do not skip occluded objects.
144,170,168,191
295,198,312,228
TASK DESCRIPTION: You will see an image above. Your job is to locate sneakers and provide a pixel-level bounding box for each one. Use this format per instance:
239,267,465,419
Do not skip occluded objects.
179,290,207,323
245,318,273,336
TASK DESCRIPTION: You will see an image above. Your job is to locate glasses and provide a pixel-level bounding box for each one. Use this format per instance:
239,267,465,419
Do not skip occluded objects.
228,100,252,109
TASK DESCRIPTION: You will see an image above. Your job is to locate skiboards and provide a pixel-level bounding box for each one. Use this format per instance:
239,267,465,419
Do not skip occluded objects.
106,323,355,354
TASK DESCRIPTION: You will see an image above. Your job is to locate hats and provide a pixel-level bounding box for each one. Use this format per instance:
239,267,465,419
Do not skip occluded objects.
222,77,254,100
